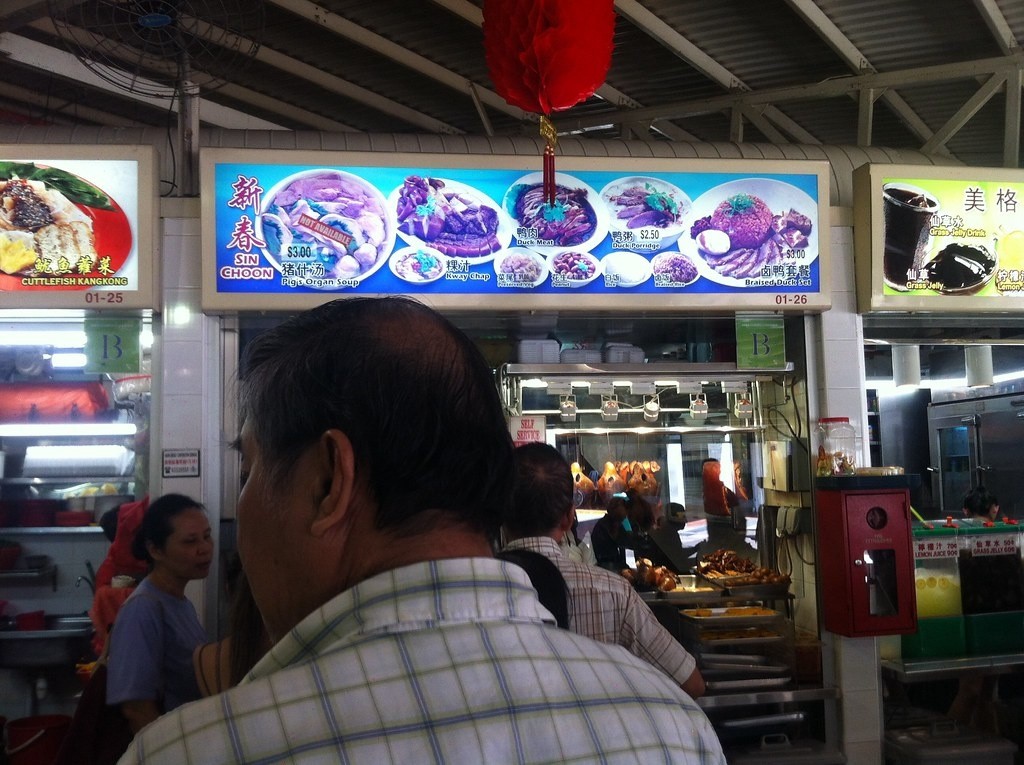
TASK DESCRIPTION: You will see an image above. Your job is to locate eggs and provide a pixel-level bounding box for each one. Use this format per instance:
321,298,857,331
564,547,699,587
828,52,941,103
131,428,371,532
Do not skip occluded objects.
696,229,730,255
744,567,782,585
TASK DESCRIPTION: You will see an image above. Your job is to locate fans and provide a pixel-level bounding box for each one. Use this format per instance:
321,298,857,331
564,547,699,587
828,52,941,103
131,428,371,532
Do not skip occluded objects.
45,1,266,197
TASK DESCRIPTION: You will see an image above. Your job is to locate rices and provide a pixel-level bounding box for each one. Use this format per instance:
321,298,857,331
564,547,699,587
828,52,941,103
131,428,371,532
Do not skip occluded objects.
709,194,774,248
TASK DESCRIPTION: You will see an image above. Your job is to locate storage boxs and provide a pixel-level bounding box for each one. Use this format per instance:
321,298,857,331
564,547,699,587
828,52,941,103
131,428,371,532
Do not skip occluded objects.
813,417,922,637
637,574,783,599
884,720,1019,765
674,605,847,765
511,339,646,364
903,518,1024,663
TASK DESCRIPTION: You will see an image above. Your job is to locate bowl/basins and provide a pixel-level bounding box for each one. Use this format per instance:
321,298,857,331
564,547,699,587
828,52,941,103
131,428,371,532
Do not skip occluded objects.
389,247,448,284
0,547,20,570
546,249,601,288
919,239,999,297
24,556,47,568
601,252,651,287
255,169,396,289
599,177,691,254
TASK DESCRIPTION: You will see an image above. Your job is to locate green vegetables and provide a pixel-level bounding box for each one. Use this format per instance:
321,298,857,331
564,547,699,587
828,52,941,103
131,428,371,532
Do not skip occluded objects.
264,198,360,275
0,161,119,212
415,194,436,217
723,193,757,218
505,183,571,223
644,183,678,215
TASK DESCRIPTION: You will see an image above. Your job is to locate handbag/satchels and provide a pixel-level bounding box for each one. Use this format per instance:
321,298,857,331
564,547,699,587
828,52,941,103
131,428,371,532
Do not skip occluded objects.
56,595,164,765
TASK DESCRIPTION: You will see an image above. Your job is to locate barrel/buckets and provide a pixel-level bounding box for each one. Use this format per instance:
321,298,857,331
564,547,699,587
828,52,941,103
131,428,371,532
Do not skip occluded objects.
4,714,72,765
94,494,135,524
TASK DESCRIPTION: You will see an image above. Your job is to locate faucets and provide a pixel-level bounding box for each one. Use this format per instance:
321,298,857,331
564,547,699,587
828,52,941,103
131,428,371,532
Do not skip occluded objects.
75,575,95,595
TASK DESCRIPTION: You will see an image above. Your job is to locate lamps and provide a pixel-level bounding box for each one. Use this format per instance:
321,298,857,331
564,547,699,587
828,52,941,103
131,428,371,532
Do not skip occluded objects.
735,393,753,418
644,394,660,422
559,394,576,422
690,392,709,420
599,394,619,422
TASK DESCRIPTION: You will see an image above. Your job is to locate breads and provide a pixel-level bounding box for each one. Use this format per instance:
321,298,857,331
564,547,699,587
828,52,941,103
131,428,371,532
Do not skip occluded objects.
35,222,98,276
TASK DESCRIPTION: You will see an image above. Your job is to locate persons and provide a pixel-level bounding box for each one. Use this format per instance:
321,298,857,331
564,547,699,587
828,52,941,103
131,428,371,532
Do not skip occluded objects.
963,486,1009,524
59,494,276,765
566,459,749,584
497,440,705,702
115,292,733,765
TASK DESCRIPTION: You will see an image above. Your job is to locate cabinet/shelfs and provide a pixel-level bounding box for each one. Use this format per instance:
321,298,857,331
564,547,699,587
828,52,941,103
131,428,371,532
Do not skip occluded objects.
0,314,150,639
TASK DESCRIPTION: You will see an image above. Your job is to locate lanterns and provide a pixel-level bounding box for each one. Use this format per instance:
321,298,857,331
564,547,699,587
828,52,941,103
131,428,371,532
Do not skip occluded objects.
485,0,616,215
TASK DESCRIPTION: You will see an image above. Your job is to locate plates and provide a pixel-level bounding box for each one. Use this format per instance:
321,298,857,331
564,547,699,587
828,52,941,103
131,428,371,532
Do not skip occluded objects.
388,177,512,265
605,346,645,363
0,499,94,526
677,178,818,288
652,252,700,285
561,350,601,364
503,172,610,256
494,248,548,287
0,162,131,291
518,339,560,364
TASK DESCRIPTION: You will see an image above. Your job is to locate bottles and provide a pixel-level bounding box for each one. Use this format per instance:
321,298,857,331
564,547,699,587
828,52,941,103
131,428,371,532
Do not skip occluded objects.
812,417,856,477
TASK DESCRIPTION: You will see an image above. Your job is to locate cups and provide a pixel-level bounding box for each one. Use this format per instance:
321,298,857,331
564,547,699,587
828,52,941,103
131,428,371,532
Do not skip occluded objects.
883,184,940,291
17,610,44,631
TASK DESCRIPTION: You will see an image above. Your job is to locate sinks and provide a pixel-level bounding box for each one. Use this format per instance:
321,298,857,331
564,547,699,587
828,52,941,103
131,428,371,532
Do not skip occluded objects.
0,616,91,638
